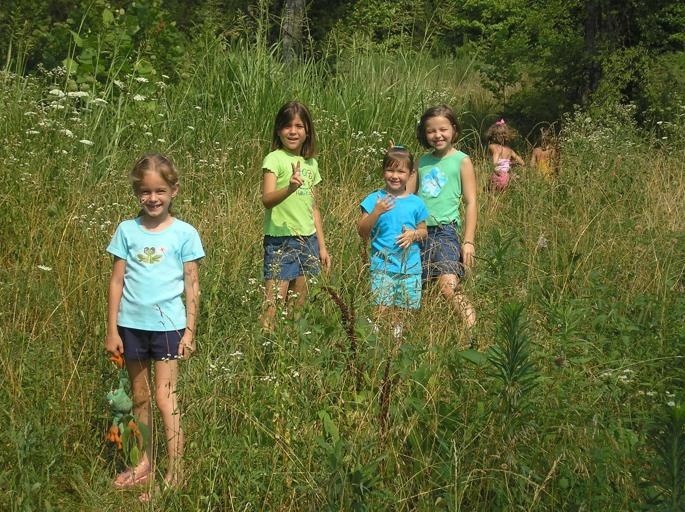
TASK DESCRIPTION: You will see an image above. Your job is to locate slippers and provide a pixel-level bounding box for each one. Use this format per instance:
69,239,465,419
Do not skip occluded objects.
137,481,188,503
112,463,156,490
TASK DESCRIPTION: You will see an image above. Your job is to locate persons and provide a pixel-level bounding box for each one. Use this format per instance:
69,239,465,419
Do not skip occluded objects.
528,125,564,178
262,99,332,330
103,154,207,503
356,141,431,337
387,104,480,327
485,119,524,195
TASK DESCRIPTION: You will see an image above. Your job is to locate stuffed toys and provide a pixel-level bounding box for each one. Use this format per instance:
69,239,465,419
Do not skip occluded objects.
105,352,145,451
